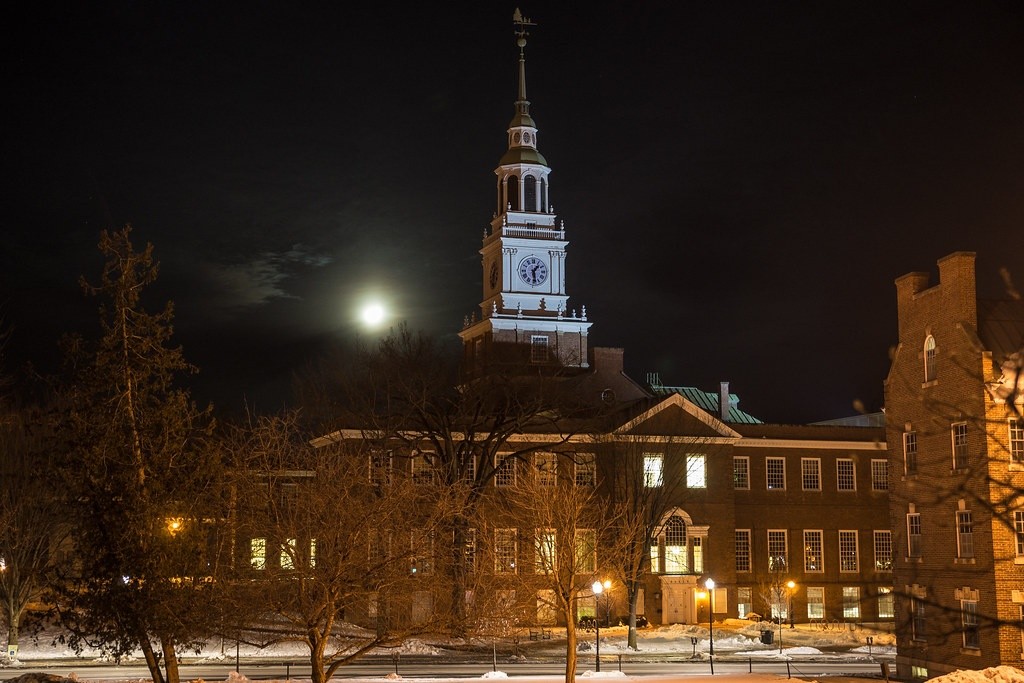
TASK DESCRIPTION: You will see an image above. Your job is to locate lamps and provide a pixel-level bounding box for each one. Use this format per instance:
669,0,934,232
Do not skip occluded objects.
653,592,660,599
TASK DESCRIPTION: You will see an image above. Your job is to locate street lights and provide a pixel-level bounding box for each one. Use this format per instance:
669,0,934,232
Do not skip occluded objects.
603,580,612,629
788,581,797,628
706,577,716,656
591,580,607,674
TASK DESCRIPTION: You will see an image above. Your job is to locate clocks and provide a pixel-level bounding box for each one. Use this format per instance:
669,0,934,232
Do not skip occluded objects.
489,261,499,290
517,254,549,288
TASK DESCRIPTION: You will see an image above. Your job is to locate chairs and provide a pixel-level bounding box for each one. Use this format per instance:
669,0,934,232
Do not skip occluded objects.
541,625,551,639
528,627,539,641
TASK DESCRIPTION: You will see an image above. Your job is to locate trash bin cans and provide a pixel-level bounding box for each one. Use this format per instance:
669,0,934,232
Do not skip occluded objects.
761,630,774,645
